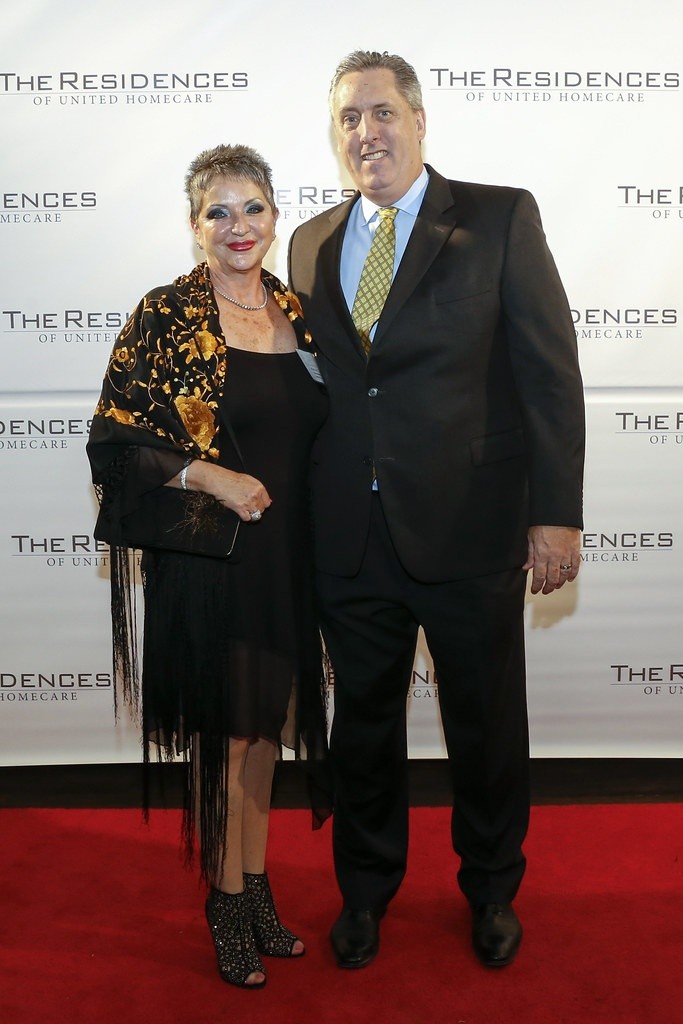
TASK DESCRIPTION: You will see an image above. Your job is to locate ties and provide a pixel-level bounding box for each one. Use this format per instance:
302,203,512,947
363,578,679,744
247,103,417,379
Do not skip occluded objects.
351,206,399,363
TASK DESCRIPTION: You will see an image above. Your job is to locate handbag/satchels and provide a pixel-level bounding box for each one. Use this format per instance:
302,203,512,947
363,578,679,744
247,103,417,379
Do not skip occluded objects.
93,482,246,564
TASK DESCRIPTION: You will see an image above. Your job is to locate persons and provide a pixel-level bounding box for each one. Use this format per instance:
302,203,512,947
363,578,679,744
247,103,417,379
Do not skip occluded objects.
287,51,586,969
85,143,332,989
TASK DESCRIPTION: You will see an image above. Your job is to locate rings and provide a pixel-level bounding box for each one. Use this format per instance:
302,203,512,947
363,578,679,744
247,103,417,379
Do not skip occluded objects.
560,565,571,570
250,509,262,521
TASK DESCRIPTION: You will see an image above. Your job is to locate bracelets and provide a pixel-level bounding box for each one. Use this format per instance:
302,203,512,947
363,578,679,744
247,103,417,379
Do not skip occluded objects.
180,457,192,491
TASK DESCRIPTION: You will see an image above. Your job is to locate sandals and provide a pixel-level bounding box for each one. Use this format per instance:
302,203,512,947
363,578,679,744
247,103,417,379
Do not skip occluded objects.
205,872,305,990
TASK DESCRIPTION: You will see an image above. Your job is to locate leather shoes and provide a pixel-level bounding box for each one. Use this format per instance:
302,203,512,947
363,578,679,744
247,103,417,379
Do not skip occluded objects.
328,900,389,968
472,897,522,970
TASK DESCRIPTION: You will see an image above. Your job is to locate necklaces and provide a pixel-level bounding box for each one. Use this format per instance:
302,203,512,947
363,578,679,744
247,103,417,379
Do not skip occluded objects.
214,282,268,310
